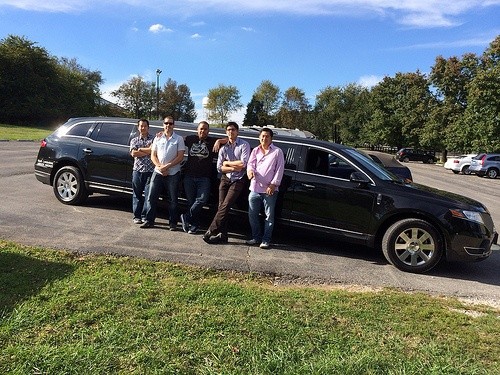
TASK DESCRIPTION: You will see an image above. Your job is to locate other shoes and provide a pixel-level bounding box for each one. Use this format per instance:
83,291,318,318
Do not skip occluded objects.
140,220,154,228
203,230,212,242
209,233,228,243
170,228,177,231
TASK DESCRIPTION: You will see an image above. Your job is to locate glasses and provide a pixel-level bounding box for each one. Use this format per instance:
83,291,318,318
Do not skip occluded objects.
163,122,173,126
199,128,209,130
227,129,236,131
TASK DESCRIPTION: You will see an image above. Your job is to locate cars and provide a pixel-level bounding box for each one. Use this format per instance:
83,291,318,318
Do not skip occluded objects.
328,151,413,184
33,115,498,274
469,153,500,179
444,154,478,175
396,148,438,164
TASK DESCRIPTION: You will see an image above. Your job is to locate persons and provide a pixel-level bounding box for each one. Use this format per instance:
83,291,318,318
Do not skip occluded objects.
245,128,284,248
130,119,156,224
140,116,185,231
156,121,228,234
202,122,250,244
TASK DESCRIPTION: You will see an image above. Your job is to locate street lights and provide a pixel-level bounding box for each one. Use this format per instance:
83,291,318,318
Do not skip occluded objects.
156,69,162,120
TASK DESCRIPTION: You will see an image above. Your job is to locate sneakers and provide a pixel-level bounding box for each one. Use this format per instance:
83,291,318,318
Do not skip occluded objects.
133,218,145,224
187,226,199,234
260,242,270,249
246,239,258,245
180,213,189,233
